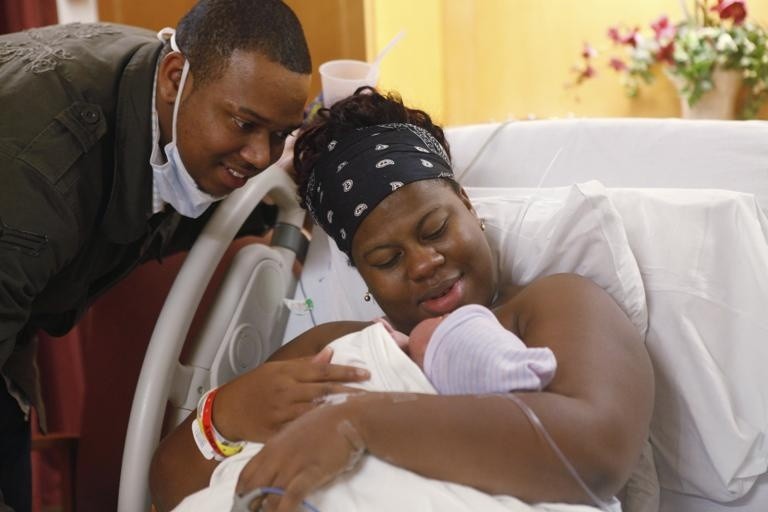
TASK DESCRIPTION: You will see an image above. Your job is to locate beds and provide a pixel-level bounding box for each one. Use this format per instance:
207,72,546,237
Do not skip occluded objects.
117,120,768,512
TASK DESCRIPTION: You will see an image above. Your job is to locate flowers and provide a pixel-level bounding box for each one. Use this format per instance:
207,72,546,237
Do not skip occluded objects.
562,1,767,116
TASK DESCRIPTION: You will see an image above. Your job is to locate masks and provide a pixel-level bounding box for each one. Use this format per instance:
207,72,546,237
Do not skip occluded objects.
149,51,230,219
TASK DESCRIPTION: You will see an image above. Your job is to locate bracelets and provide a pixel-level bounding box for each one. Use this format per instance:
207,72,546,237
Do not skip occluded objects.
193,388,253,466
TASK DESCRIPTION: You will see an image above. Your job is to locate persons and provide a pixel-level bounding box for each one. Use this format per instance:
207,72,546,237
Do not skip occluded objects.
147,85,664,512
317,302,561,407
0,0,311,511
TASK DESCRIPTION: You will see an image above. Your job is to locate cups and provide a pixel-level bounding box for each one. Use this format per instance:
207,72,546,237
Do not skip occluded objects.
317,59,377,118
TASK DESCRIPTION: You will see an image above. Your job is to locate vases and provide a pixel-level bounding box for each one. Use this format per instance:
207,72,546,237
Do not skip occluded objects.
664,71,743,121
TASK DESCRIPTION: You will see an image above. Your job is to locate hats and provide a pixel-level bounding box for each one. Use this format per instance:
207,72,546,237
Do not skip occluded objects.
423,302,556,394
